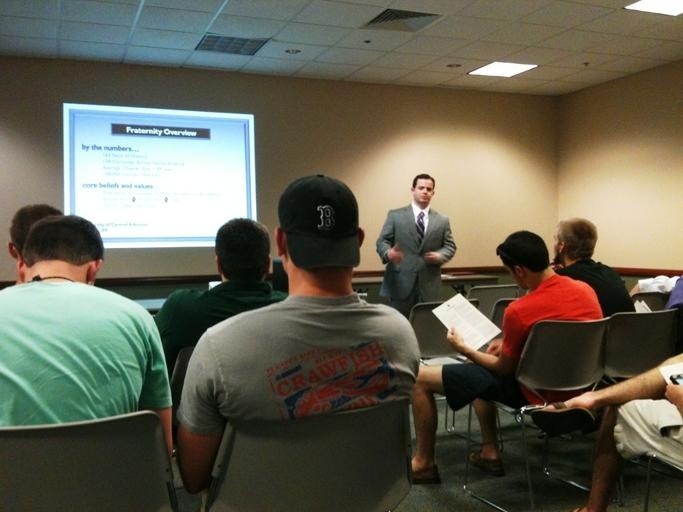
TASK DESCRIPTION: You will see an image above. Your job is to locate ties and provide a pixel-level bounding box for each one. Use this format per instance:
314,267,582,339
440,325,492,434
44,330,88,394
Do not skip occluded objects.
416,212,424,244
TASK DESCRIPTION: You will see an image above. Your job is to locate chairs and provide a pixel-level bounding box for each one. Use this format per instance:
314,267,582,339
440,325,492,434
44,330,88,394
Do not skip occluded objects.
465,283,523,310
169,347,194,490
1,410,180,511
203,396,411,511
603,306,678,382
405,297,482,432
615,446,683,512
463,316,610,511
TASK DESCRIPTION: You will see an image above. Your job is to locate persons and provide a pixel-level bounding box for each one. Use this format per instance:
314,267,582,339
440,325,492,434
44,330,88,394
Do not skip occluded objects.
411,231,604,484
155,218,287,383
530,351,683,512
7,205,65,284
550,217,683,320
375,174,457,322
0,215,174,512
175,174,420,511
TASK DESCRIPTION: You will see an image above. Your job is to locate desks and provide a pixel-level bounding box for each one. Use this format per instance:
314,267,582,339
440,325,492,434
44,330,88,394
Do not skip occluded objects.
352,274,502,300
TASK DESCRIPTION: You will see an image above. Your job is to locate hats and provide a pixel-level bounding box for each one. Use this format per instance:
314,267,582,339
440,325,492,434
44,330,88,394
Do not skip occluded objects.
278,174,360,269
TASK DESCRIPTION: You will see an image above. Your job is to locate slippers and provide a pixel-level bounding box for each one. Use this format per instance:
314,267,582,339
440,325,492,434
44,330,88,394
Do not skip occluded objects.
531,402,596,435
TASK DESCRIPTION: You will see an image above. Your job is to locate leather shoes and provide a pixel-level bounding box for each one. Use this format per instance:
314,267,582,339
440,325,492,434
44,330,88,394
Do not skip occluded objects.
411,464,440,484
468,451,502,472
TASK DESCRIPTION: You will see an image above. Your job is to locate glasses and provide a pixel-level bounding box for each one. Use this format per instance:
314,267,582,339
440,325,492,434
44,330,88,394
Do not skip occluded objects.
497,244,519,264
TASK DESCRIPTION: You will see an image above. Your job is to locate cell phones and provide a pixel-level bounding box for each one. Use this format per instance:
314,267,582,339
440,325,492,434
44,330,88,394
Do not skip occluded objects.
670,374,683,385
